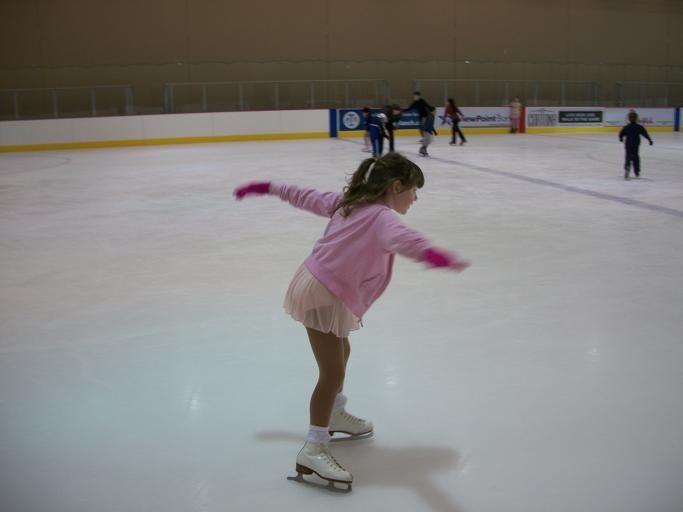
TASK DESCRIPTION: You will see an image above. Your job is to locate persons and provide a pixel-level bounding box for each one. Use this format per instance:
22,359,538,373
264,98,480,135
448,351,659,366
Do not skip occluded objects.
232,152,473,482
510,96,522,133
361,91,467,158
619,113,653,178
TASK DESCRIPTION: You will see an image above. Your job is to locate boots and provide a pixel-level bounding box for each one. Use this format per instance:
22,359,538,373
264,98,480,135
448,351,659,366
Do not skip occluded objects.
296,440,353,483
328,407,373,435
